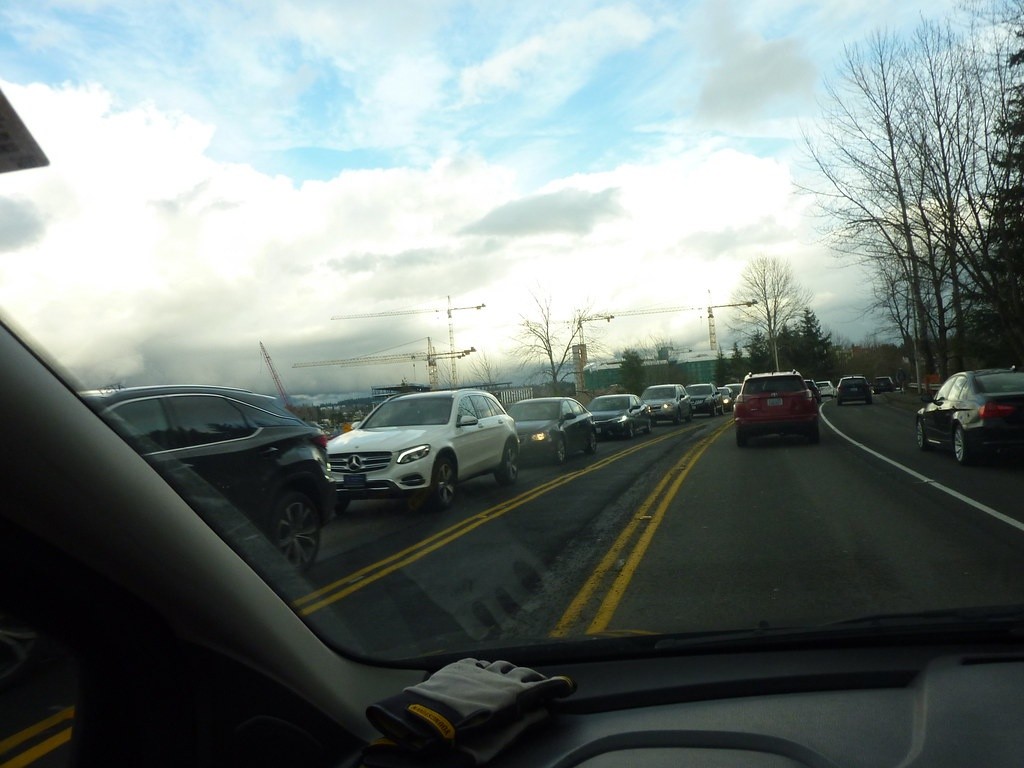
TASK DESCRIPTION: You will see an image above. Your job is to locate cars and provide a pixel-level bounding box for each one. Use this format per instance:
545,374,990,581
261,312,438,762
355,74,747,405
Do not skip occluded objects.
718,386,734,412
586,394,653,443
915,366,1024,463
817,381,838,398
725,383,744,400
504,394,597,468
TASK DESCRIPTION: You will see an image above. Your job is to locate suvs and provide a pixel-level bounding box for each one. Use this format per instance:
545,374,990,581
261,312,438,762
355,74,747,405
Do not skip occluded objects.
641,382,693,424
325,386,522,510
685,383,723,417
73,383,337,576
836,376,873,405
803,378,822,403
733,368,819,446
876,376,895,392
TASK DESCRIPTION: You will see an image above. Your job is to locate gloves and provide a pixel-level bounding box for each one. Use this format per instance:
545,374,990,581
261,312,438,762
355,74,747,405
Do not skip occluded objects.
365,658,576,748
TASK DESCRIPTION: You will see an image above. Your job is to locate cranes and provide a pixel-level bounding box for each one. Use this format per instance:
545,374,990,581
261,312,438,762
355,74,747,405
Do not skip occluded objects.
255,341,297,417
330,293,486,389
565,306,615,396
604,289,760,353
290,336,477,392
